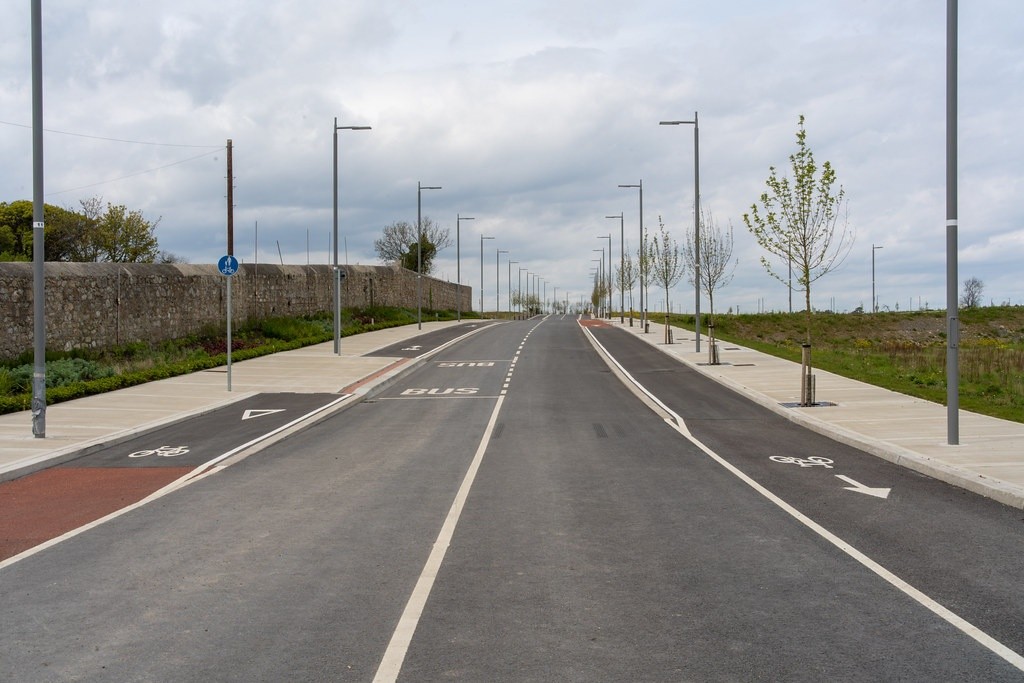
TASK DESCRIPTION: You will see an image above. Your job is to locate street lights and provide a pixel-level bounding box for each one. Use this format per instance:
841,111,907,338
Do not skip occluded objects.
518,267,528,320
597,233,611,319
481,234,495,320
333,117,373,353
605,211,625,324
497,249,509,318
873,244,883,312
527,272,544,318
618,179,643,328
457,214,475,322
659,111,700,353
417,181,442,330
509,260,519,319
589,247,604,318
544,281,550,314
